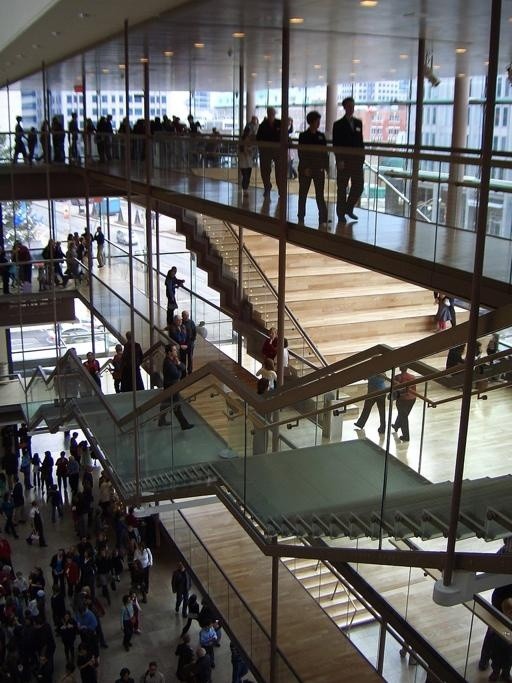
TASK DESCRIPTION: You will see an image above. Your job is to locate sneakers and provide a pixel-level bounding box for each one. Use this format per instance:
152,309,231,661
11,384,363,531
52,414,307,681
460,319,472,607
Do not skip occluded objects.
392,424,398,432
399,436,409,441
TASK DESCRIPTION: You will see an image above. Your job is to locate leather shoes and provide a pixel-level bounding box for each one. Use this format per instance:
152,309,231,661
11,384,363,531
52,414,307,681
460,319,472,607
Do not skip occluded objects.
319,210,358,223
181,423,195,430
158,421,172,426
